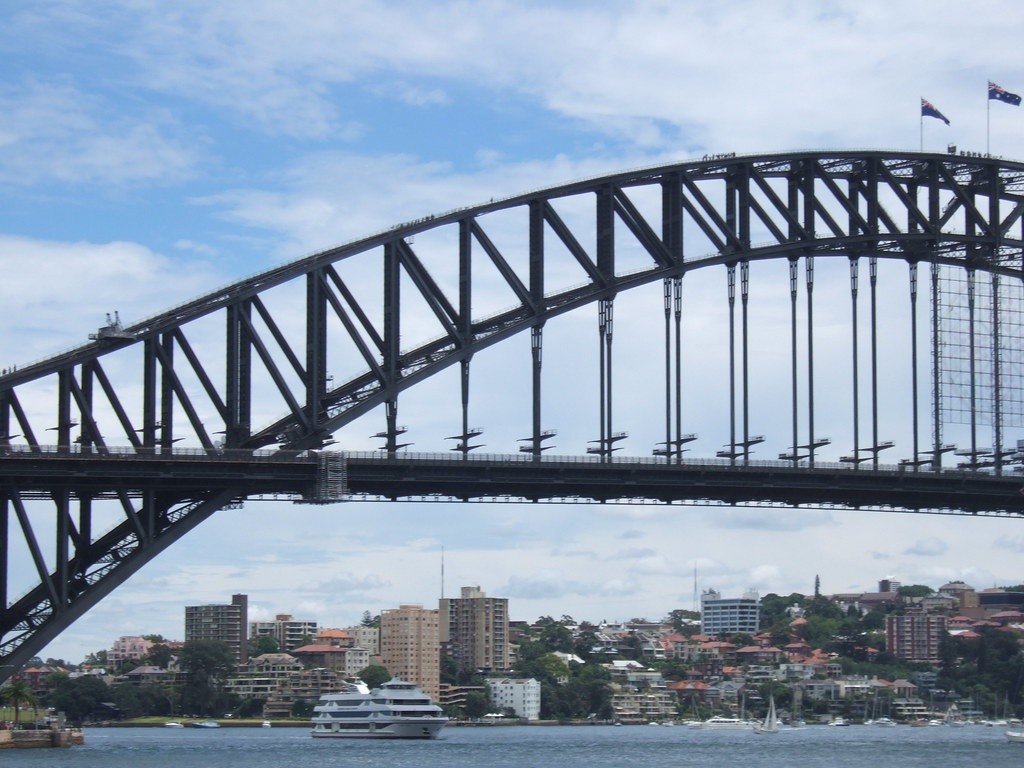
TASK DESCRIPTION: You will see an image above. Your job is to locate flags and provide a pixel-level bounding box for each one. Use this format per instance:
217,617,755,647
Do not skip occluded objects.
921,97,950,127
988,80,1022,106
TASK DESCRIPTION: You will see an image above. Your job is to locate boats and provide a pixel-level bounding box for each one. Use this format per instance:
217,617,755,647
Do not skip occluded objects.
309,678,449,743
663,721,675,727
1005,732,1024,744
262,720,272,728
863,686,897,726
615,722,623,726
650,721,657,726
165,722,186,729
908,702,1024,728
825,715,850,727
191,721,221,729
683,696,806,733
